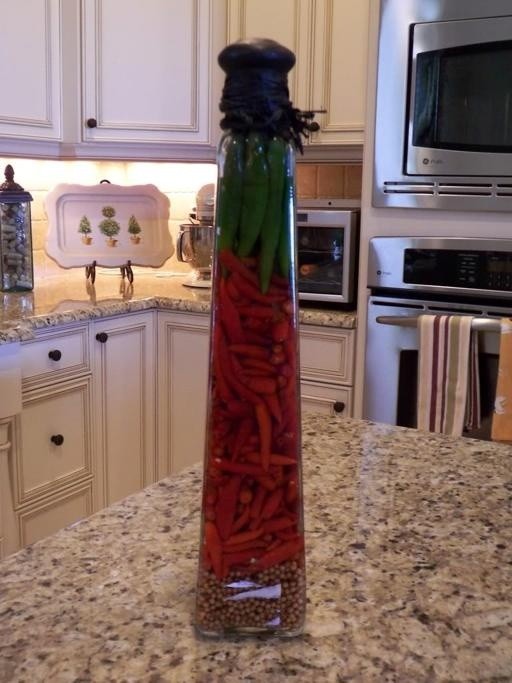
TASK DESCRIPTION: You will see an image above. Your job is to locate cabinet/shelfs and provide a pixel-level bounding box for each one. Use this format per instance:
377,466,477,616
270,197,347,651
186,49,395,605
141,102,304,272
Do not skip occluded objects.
152,298,212,481
214,0,378,155
297,311,368,417
0,303,162,566
1,0,213,164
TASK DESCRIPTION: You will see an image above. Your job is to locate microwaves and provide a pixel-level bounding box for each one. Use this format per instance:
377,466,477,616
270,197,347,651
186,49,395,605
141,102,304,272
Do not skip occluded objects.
404,15,512,175
295,207,360,309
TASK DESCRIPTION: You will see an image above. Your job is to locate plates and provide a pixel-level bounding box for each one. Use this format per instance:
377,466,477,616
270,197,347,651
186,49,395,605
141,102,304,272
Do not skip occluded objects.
43,182,177,268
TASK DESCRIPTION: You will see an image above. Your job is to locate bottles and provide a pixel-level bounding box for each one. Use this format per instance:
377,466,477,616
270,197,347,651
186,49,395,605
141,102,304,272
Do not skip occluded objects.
298,262,329,280
191,32,311,640
1,163,36,294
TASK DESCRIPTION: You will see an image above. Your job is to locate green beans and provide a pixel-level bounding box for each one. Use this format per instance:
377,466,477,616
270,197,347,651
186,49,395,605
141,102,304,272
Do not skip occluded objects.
199,562,305,630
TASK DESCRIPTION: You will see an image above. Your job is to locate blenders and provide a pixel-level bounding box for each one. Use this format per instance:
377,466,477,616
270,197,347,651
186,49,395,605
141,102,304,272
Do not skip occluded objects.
175,183,215,288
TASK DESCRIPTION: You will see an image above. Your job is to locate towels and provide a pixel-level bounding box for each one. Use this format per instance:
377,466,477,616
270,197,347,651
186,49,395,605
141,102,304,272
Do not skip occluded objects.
489,314,511,444
417,312,481,436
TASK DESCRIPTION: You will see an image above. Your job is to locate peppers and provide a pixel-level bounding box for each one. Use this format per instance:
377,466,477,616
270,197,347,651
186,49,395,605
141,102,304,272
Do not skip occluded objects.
201,133,304,580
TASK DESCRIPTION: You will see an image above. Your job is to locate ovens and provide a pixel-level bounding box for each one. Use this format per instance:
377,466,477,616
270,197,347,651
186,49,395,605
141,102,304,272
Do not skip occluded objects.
361,234,512,446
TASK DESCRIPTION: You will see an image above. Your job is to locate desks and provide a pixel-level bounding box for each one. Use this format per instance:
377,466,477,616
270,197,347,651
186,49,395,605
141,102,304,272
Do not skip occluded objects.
0,410,511,683
